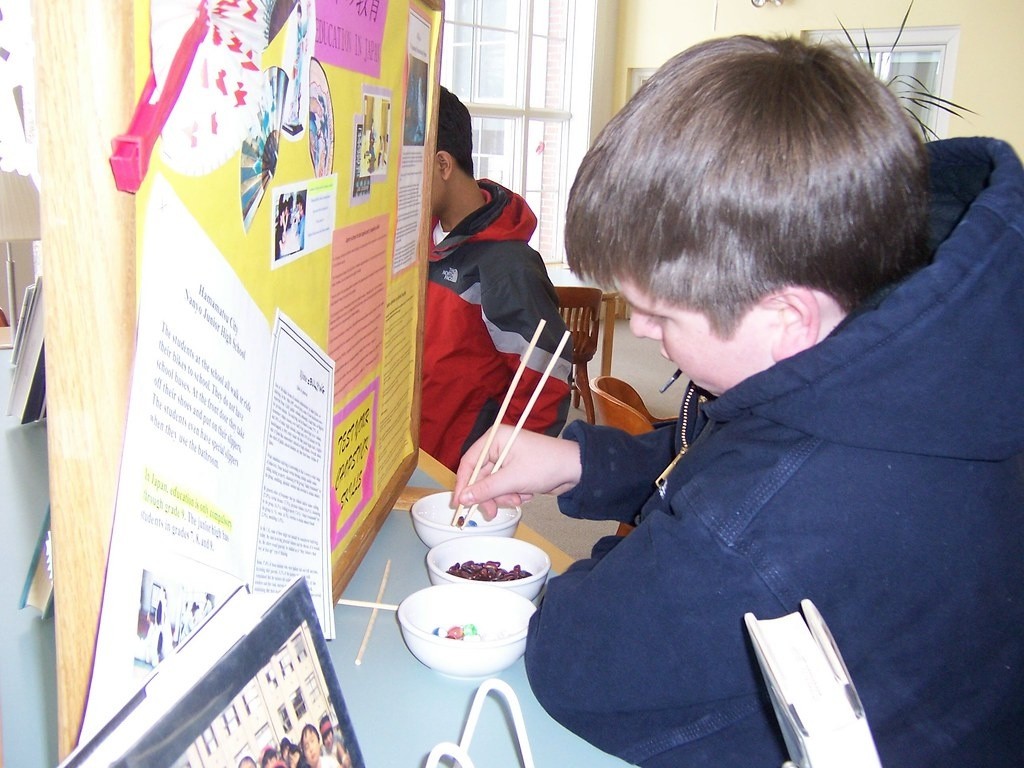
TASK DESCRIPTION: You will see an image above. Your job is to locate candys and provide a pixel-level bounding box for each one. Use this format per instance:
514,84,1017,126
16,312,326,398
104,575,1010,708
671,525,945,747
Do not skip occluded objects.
457,516,464,526
469,521,476,527
447,561,531,581
433,624,481,641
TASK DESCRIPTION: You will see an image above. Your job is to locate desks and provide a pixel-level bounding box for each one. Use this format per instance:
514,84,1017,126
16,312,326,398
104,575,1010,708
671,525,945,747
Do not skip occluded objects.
545,267,621,377
0,417,644,768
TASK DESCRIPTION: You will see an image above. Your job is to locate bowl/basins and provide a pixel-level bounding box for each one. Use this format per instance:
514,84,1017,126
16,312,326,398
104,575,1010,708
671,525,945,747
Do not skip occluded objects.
412,492,522,548
427,536,551,601
397,583,538,677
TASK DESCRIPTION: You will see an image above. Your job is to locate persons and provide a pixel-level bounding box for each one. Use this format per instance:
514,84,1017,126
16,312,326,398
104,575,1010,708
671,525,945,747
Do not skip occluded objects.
417,87,573,474
275,193,306,262
450,32,1024,768
238,715,352,768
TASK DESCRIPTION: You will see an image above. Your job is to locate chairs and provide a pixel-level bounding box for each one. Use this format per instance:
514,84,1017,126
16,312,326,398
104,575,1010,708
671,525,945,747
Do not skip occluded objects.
554,286,604,424
589,376,678,537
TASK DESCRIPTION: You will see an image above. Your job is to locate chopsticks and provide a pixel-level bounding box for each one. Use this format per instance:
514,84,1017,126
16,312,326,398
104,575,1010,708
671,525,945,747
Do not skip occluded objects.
451,319,571,530
337,559,400,666
